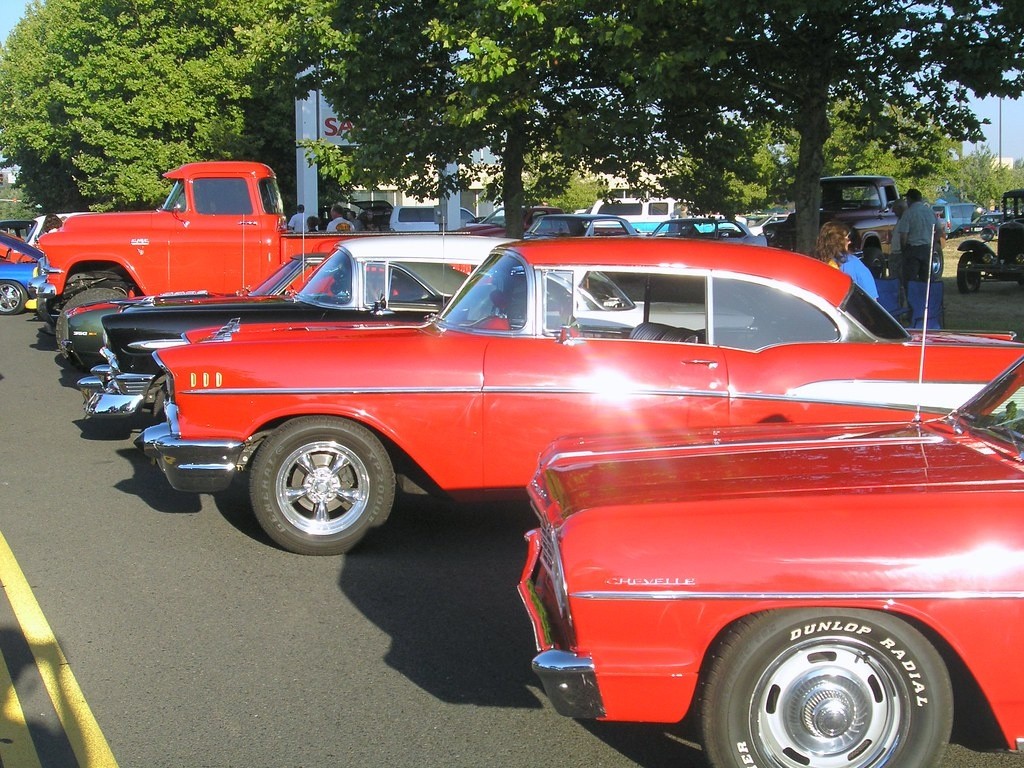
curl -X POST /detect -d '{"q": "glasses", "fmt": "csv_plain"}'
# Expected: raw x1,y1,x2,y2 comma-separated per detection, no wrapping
844,236,850,242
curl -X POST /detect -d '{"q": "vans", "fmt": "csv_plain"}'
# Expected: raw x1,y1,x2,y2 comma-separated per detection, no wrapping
581,196,748,233
348,200,476,232
931,203,984,235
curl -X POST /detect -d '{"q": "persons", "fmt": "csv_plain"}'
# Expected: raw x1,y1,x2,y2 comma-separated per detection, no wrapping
288,205,309,233
735,213,747,225
887,200,907,278
680,206,688,218
307,216,320,232
344,208,380,231
542,202,548,206
898,188,942,311
44,214,62,233
971,207,982,223
814,221,878,303
326,205,355,231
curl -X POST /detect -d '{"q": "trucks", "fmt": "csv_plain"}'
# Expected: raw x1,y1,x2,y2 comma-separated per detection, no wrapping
28,161,470,339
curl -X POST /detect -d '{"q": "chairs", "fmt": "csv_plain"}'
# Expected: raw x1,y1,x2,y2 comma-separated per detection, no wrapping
629,322,672,341
660,327,701,344
874,280,946,329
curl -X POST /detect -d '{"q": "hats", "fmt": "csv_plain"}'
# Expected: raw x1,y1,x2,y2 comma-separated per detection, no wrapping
903,189,923,202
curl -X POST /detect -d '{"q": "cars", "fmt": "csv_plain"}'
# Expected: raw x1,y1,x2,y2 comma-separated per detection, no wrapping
514,360,1024,768
45,234,538,431
749,214,788,236
964,213,1019,241
451,207,566,239
524,214,639,239
762,176,944,282
0,212,100,316
646,219,768,248
132,237,1018,557
